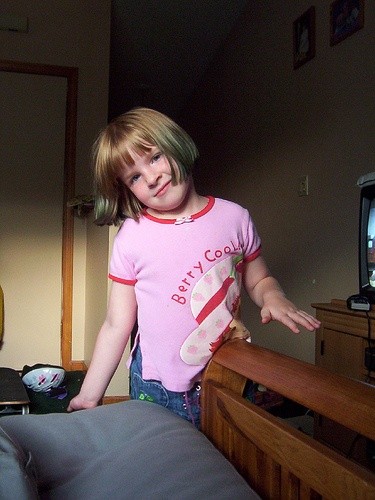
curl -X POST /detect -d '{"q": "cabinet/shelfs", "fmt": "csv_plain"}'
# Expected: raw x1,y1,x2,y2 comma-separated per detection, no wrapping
311,303,375,387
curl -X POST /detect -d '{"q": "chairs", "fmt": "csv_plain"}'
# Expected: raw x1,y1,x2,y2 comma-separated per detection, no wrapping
0,287,30,415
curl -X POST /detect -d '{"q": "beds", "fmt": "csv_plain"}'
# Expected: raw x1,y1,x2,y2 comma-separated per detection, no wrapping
0,339,375,500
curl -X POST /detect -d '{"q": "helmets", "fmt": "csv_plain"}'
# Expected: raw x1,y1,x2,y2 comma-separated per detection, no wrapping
21,364,66,393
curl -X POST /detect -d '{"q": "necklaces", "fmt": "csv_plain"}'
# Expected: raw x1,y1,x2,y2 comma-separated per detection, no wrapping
66,108,322,431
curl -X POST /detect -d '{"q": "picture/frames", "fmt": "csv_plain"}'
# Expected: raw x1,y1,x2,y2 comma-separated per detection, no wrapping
329,0,365,46
291,6,315,69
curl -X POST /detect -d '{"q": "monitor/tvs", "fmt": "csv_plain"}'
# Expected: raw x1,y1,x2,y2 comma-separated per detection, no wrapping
357,183,375,304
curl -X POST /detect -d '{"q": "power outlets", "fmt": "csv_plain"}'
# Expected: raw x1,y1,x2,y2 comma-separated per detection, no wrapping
297,175,308,196
